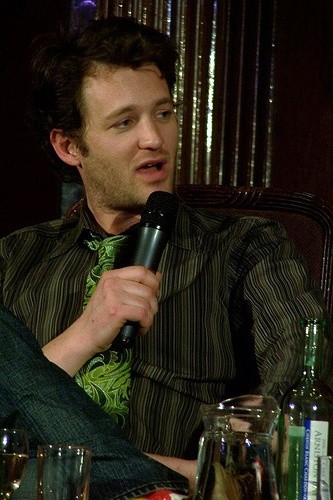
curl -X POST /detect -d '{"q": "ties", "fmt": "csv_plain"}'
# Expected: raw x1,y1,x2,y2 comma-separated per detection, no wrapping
72,231,133,429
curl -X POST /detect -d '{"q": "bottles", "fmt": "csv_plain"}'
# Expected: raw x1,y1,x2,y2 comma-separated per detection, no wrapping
277,317,333,500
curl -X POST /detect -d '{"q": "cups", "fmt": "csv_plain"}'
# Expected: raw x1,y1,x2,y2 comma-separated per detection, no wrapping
36,444,90,500
315,455,333,500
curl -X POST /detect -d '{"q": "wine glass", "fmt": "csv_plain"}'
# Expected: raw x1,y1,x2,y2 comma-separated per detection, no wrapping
0,427,30,500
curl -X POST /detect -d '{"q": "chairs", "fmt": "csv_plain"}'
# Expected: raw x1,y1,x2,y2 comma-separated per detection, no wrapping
61,185,333,317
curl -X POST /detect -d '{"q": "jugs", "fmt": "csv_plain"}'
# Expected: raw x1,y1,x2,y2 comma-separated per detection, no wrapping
194,394,282,500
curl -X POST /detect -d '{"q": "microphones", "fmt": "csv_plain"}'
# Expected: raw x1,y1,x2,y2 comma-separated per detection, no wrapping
112,190,178,348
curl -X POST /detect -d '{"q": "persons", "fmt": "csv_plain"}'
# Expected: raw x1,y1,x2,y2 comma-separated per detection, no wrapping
0,17,330,500
